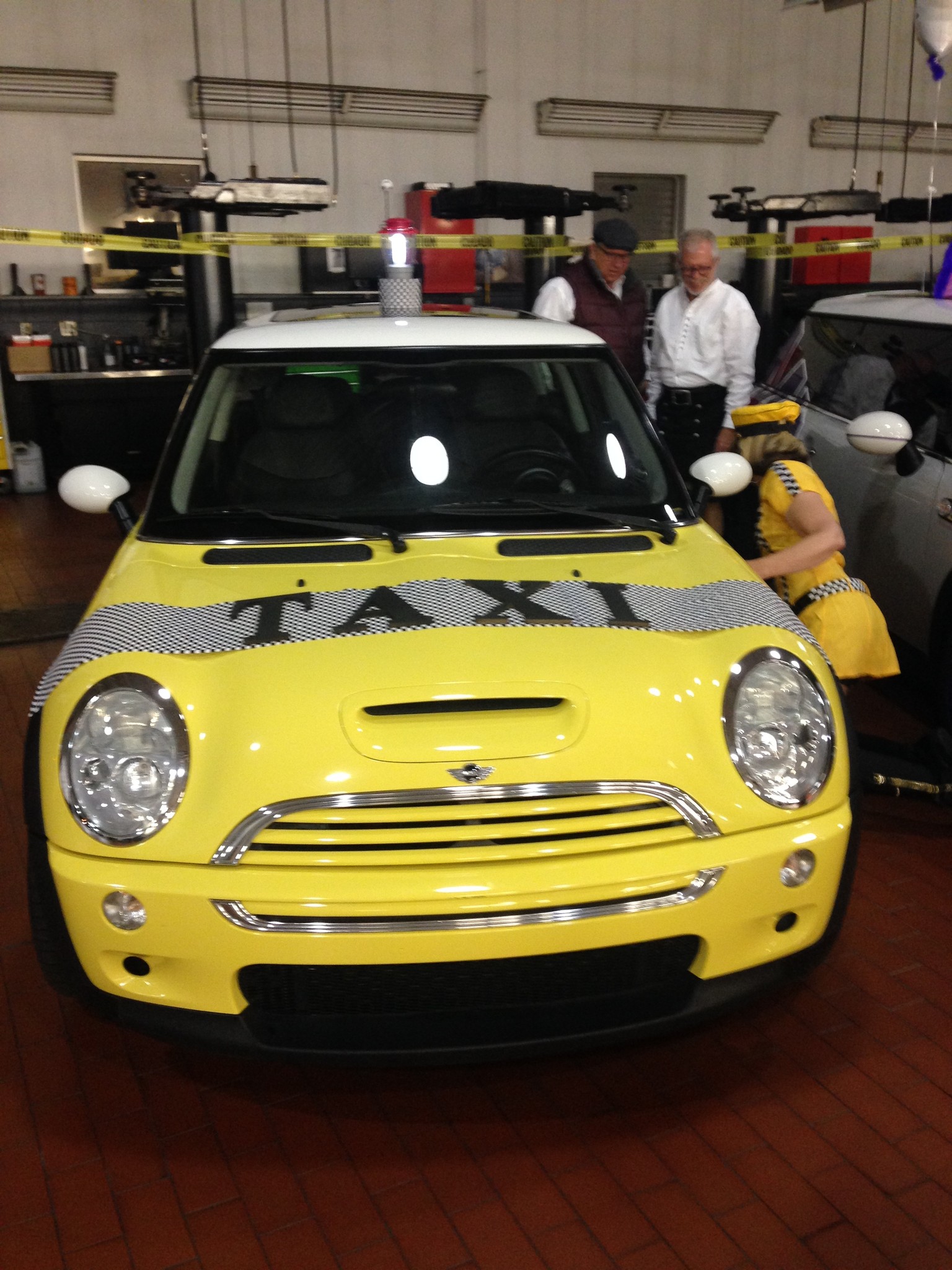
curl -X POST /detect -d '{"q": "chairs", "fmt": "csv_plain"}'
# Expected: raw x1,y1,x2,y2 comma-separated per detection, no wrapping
436,367,583,493
222,370,365,518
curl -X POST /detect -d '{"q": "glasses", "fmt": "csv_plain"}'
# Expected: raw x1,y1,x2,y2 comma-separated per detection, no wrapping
679,261,715,277
595,242,636,261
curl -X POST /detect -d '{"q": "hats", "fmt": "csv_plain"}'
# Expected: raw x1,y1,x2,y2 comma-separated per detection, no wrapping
593,218,638,252
731,398,802,439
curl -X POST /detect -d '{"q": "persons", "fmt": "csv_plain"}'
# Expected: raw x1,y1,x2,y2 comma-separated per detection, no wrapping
823,330,951,460
526,216,653,406
727,396,904,798
640,228,764,539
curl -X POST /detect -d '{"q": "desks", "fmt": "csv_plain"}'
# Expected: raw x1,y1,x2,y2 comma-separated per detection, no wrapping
13,367,196,486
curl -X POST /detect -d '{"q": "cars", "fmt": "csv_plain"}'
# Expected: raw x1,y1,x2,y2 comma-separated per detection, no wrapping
24,307,856,1058
756,290,952,780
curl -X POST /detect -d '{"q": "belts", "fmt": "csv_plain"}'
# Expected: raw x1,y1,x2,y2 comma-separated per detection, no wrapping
660,384,725,406
